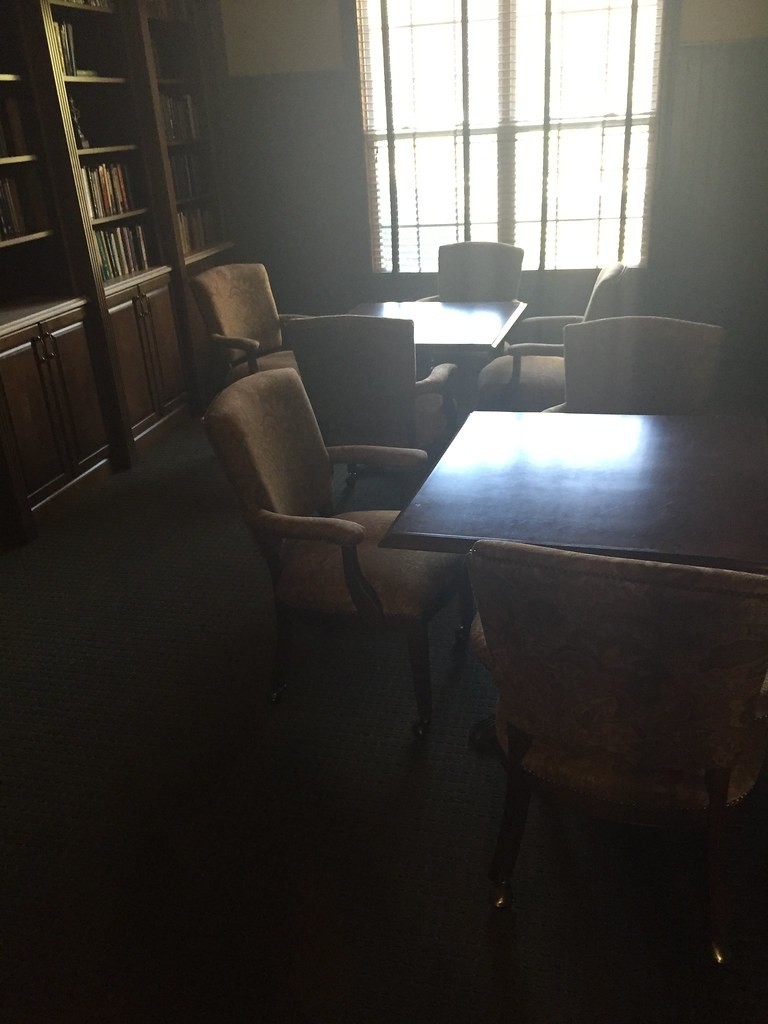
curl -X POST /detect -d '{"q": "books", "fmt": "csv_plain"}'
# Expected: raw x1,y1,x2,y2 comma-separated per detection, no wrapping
0,177,27,237
53,22,76,78
81,162,148,281
159,94,217,254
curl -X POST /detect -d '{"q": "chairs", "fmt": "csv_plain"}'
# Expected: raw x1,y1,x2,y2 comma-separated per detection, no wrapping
282,315,462,488
466,539,768,965
542,316,724,415
203,366,472,760
190,262,314,394
476,261,632,410
413,240,524,384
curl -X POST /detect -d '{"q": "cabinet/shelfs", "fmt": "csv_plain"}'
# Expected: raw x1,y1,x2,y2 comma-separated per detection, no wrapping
0,0,234,554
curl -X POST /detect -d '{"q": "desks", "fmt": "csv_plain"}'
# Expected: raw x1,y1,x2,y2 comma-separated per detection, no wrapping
345,301,529,426
379,408,768,751
